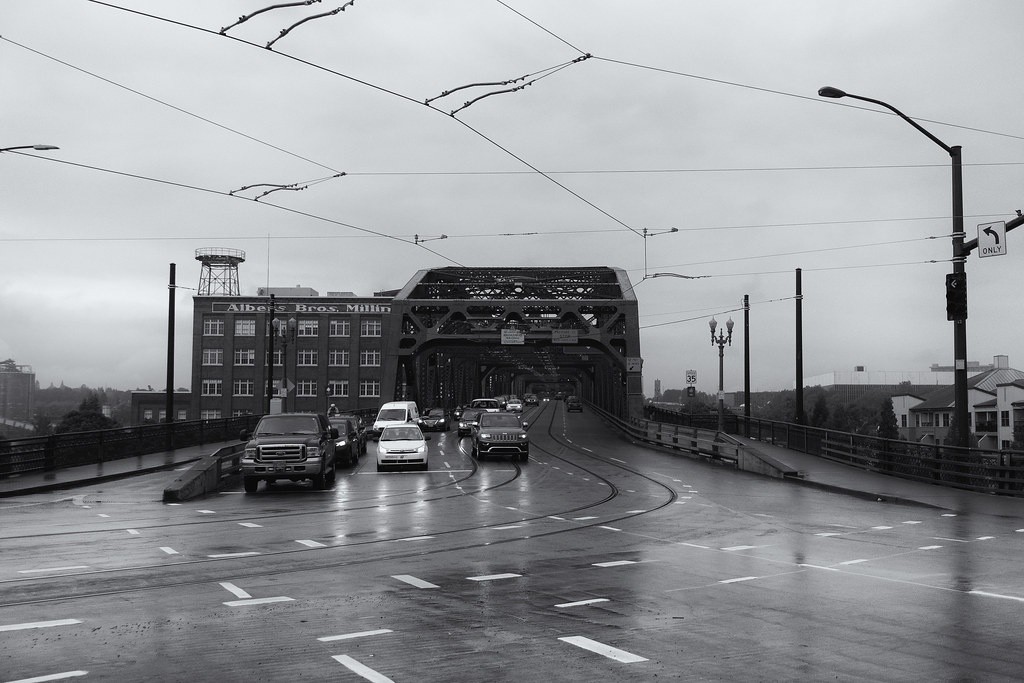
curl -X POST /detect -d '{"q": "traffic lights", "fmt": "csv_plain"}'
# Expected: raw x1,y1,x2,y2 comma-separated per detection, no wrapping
944,273,968,321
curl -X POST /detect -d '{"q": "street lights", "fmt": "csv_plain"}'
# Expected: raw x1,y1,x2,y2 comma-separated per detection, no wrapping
708,314,734,437
817,85,971,490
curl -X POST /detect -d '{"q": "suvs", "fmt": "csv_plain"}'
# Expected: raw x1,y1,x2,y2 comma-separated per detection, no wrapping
467,411,530,461
239,411,339,493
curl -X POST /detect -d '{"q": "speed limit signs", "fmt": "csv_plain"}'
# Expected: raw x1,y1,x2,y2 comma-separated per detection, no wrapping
685,370,697,384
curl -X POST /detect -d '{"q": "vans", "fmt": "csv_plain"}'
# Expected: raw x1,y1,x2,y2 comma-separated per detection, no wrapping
373,401,420,437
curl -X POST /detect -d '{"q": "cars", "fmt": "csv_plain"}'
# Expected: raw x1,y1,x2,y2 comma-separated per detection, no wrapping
420,389,583,434
328,412,368,469
373,424,431,472
